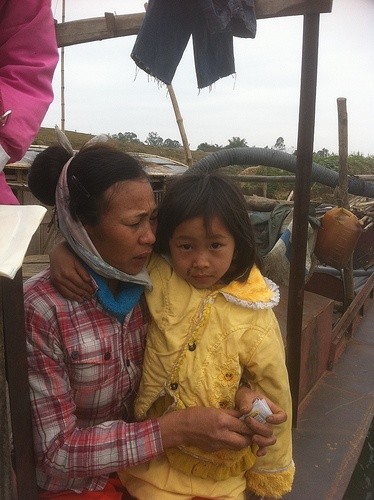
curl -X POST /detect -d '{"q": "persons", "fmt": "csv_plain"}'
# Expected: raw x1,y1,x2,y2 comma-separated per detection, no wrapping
0,0,59,205
23,125,288,500
48,171,296,500
